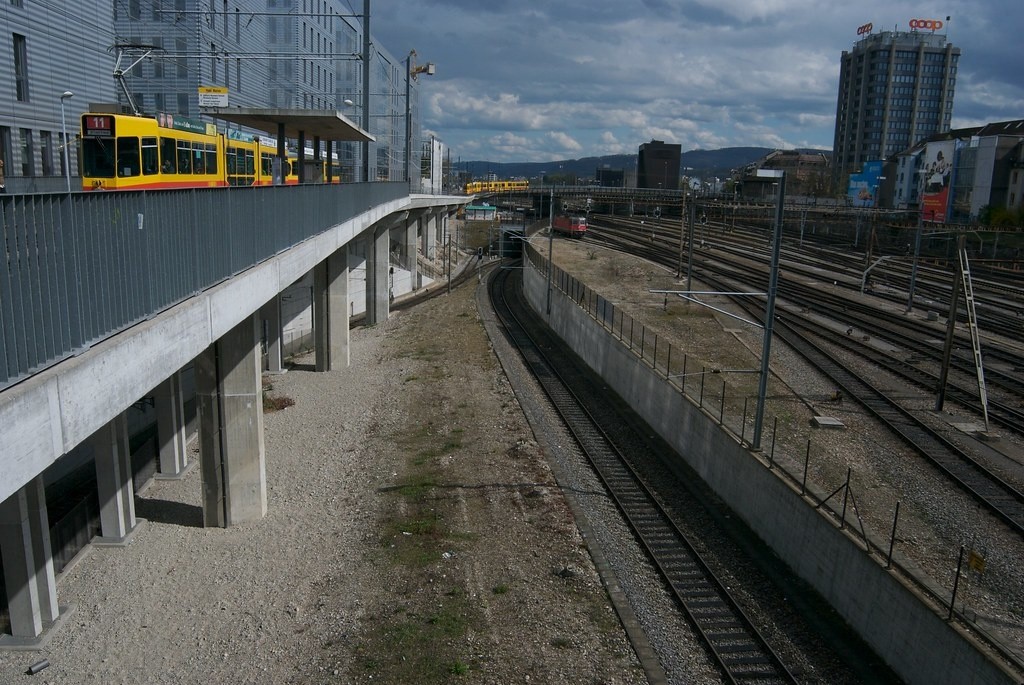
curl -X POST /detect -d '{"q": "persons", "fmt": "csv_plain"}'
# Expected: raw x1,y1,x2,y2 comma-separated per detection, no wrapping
923,151,952,194
162,157,205,174
905,244,911,257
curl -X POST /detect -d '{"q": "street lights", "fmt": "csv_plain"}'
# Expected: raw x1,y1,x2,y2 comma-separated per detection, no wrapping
60,91,73,192
864,175,888,283
905,168,928,310
539,171,547,220
748,168,787,452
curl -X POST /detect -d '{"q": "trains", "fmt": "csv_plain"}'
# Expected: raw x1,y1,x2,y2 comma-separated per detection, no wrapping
458,177,530,196
552,214,588,240
79,44,341,193
